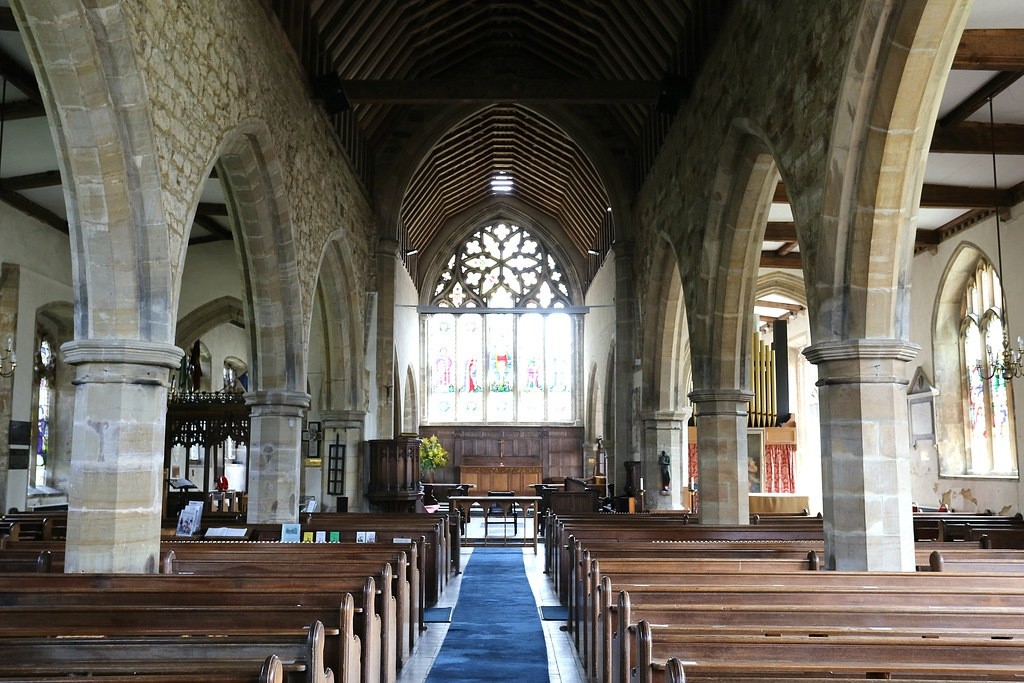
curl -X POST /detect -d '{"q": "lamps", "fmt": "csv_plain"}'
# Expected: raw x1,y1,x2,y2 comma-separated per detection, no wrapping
976,96,1024,380
0,339,18,378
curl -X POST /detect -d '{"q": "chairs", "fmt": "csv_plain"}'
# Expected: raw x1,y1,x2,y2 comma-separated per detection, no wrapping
484,490,517,535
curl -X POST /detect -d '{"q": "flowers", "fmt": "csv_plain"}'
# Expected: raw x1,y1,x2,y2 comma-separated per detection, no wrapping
419,437,450,474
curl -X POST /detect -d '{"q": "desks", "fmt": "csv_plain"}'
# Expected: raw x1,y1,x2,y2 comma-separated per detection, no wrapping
448,495,542,555
749,493,811,513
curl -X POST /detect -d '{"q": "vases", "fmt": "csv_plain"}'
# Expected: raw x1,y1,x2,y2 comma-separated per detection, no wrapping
421,466,434,484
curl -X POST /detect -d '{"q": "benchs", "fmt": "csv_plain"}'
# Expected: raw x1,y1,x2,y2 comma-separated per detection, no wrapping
529,477,1024,683
0,483,475,683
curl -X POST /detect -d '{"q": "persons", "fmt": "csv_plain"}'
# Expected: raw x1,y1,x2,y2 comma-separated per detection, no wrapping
215,473,228,492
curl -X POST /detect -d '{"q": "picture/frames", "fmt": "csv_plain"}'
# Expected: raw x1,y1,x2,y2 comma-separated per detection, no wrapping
747,428,765,495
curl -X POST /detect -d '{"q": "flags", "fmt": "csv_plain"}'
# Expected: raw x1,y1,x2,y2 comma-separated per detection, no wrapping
239,371,248,392
191,339,203,392
